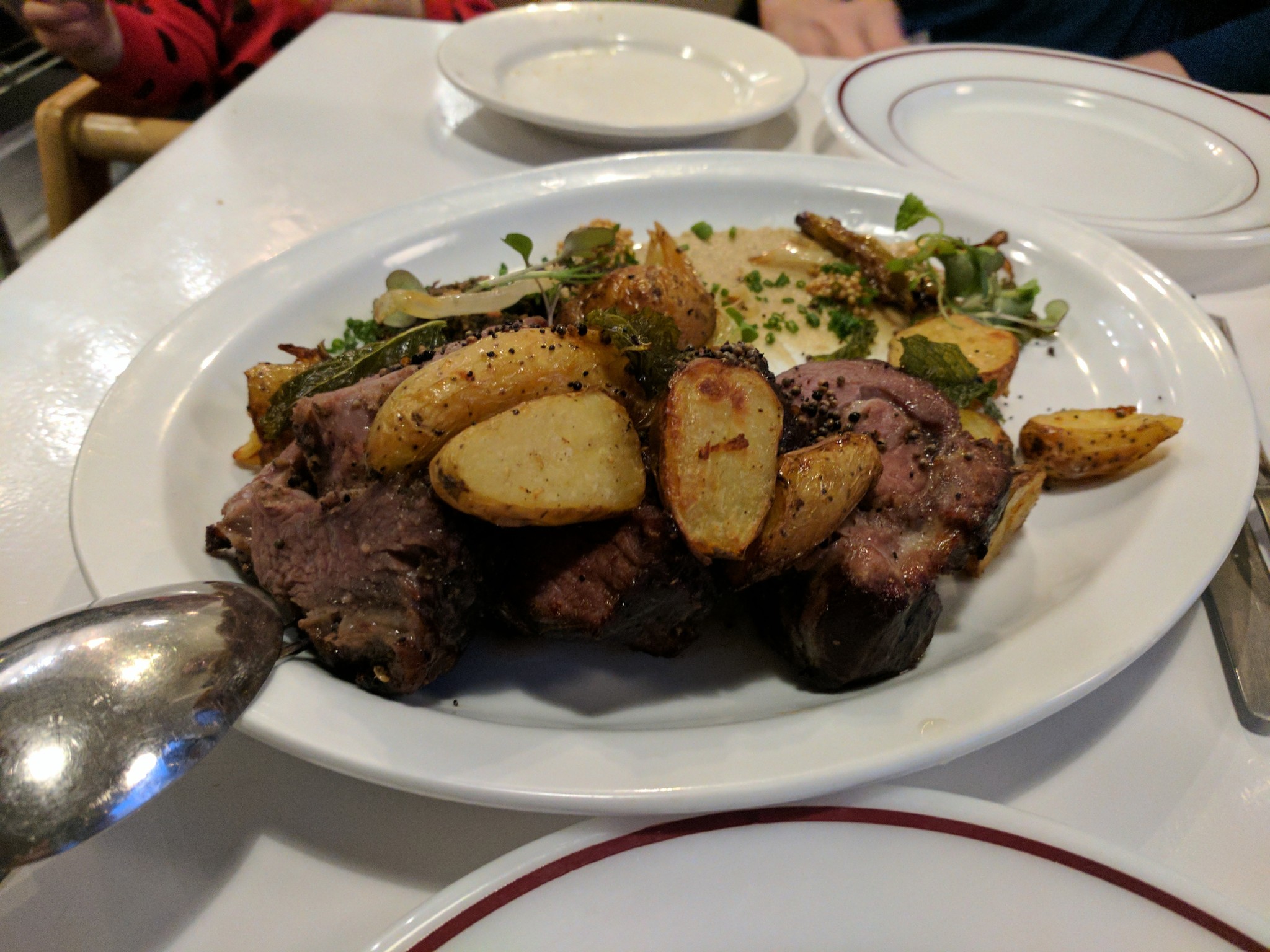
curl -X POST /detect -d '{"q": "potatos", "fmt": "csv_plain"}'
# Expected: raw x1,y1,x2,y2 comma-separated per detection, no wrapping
230,220,1186,582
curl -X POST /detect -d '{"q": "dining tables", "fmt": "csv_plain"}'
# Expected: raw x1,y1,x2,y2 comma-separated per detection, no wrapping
0,10,1270,952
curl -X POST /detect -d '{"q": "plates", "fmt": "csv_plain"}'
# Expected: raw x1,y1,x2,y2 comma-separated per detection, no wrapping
64,145,1263,814
820,40,1270,250
437,1,810,141
364,777,1270,952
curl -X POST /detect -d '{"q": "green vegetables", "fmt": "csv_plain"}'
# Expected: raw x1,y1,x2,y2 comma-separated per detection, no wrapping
256,191,1071,419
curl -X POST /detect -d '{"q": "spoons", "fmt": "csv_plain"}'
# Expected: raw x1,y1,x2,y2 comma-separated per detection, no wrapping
0,578,285,885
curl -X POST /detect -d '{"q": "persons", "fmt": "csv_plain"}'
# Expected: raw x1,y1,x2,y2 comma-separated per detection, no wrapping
22,0,504,123
756,1,1270,98
